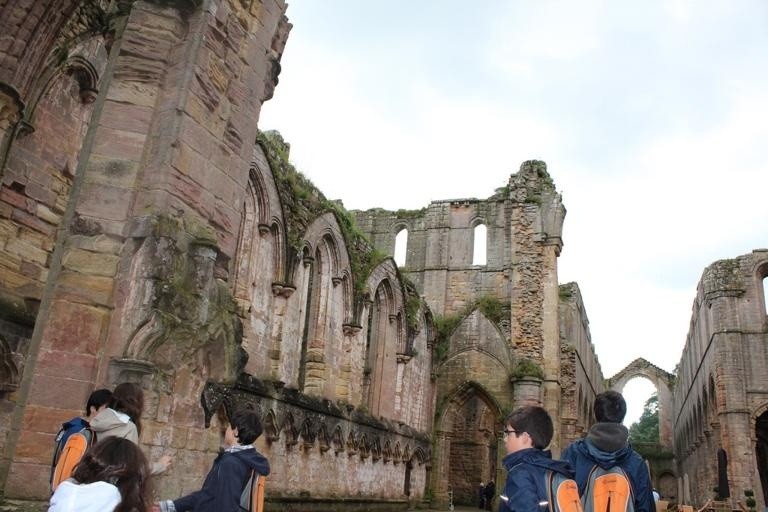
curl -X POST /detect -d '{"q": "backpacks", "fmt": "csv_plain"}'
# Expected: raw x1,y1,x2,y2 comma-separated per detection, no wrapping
580,462,637,511
545,468,583,511
239,469,265,512
50,427,97,495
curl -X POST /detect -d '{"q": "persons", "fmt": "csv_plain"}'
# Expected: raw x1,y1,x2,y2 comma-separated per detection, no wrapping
496,405,576,512
89,382,173,477
47,435,156,512
479,480,495,511
145,409,270,512
561,390,656,512
50,388,112,496
652,488,661,507
